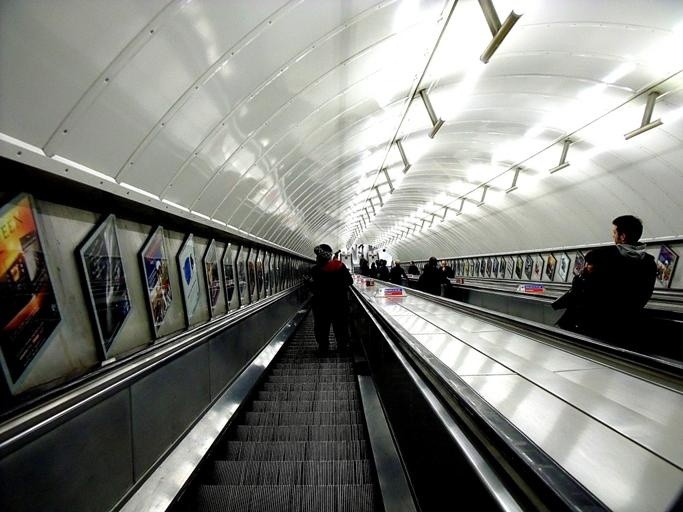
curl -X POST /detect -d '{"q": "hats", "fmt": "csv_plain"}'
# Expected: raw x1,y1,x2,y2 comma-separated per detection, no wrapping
313,244,332,259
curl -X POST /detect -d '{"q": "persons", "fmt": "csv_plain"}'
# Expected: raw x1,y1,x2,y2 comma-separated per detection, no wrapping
571,214,657,344
357,254,454,299
551,248,605,331
305,244,353,356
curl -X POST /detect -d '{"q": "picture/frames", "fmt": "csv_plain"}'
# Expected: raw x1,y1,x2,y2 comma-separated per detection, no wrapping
447,245,679,290
